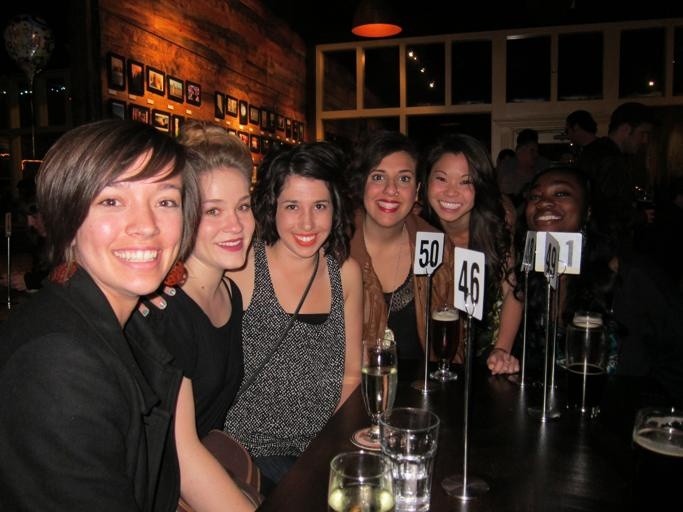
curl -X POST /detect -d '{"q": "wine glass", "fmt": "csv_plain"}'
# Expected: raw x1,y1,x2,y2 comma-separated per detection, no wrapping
355,337,397,443
428,305,459,384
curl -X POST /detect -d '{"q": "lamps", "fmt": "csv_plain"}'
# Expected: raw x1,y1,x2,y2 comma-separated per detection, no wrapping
348,0,405,45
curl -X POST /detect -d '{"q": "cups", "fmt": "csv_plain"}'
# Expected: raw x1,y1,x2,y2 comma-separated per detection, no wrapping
377,408,440,509
326,450,395,512
558,308,682,511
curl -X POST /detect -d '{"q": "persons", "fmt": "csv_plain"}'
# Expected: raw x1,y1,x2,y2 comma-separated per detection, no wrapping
418,129,527,376
122,124,264,512
0,113,199,511
137,138,366,503
0,169,62,292
346,130,462,385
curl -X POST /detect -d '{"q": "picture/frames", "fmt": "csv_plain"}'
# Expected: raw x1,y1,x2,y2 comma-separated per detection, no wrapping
99,49,307,192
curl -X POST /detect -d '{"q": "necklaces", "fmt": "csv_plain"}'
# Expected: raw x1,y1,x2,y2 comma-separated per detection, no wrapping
364,223,406,352
